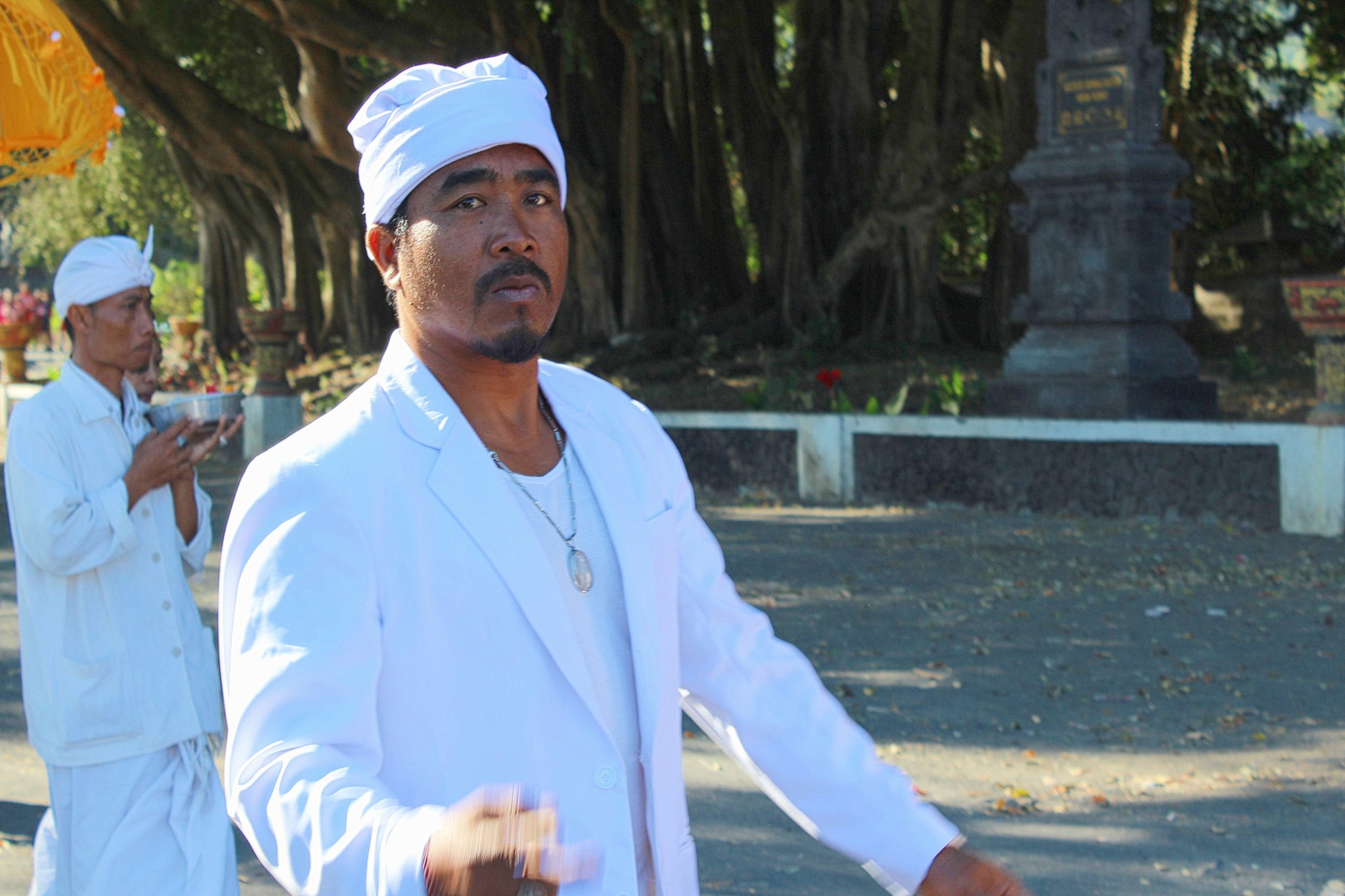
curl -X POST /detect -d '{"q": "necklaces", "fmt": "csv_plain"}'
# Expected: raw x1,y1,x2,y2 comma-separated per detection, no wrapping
485,393,592,589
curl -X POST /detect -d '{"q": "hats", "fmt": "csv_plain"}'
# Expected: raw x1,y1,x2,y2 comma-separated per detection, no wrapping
348,53,567,226
53,225,155,319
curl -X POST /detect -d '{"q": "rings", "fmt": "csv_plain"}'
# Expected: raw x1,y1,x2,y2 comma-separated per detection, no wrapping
220,435,228,446
177,433,187,447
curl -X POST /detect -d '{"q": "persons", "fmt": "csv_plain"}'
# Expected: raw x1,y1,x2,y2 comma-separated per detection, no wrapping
6,224,237,896
219,54,1026,896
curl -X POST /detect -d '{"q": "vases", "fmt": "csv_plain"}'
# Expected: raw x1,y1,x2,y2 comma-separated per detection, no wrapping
0,324,36,384
237,310,307,397
1281,277,1344,425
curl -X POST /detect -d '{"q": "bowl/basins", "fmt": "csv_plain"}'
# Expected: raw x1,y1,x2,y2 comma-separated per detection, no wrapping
142,392,246,434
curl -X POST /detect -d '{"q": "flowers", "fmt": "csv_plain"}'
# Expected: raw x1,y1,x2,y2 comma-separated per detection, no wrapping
0,289,51,325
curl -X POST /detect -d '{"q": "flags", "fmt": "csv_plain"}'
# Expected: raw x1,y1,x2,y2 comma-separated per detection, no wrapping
0,0,124,188
